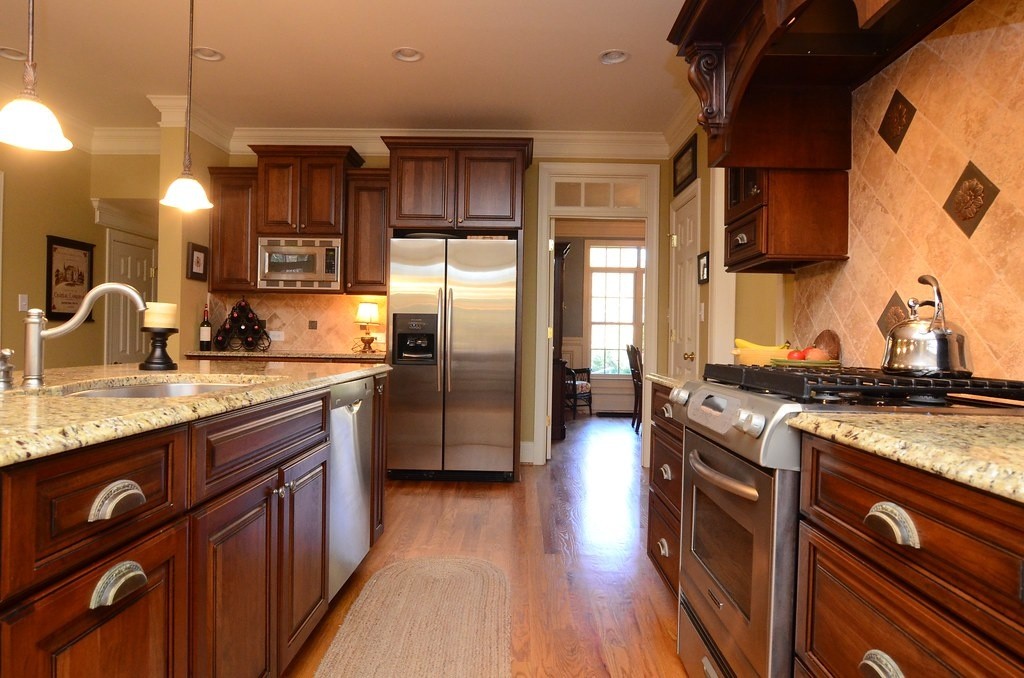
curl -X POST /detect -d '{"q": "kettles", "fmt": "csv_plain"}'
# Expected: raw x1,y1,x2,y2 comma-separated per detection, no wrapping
881,275,951,376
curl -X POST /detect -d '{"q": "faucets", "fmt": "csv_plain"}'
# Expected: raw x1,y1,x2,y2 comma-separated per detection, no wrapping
23,281,149,387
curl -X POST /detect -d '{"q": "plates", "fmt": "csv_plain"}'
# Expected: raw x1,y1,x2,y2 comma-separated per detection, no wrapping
771,359,841,368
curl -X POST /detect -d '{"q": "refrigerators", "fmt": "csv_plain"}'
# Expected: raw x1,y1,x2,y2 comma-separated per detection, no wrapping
386,238,517,482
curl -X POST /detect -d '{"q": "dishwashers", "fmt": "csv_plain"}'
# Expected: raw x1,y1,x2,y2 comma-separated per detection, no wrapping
326,376,375,603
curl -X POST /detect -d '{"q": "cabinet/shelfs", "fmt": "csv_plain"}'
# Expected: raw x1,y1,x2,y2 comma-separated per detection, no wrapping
204,136,535,295
793,434,1024,678
726,168,849,274
647,382,684,602
0,373,329,678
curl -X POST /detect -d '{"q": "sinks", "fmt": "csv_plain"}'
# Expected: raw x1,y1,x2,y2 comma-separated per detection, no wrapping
42,374,277,399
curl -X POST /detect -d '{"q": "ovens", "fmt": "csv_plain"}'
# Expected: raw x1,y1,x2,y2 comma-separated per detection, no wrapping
676,426,801,678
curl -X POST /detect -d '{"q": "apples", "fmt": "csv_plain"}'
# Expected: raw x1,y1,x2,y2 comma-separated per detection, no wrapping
787,346,814,360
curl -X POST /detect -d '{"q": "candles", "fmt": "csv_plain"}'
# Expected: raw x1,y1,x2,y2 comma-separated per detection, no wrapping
144,302,178,329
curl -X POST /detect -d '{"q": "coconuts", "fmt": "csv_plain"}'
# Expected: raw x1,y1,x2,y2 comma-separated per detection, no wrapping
813,329,840,360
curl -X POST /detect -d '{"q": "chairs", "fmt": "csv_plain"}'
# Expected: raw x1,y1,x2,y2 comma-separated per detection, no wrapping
565,368,593,420
627,345,643,432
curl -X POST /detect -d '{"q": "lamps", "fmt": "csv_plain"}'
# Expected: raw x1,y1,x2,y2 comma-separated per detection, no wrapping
0,0,72,152
159,0,214,212
354,301,380,353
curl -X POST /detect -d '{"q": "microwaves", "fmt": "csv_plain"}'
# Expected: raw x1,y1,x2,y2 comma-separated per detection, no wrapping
258,238,341,291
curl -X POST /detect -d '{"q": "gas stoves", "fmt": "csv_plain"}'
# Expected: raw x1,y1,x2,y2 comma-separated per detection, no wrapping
668,364,1024,471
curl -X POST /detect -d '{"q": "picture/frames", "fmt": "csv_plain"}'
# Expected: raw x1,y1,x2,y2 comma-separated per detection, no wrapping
186,242,209,281
697,250,709,284
673,133,697,196
45,235,96,322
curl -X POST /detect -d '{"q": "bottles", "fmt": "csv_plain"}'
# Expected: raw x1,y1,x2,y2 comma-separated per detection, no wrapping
199,304,213,352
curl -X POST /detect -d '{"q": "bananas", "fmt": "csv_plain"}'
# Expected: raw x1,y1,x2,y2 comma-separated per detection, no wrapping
734,339,791,350
